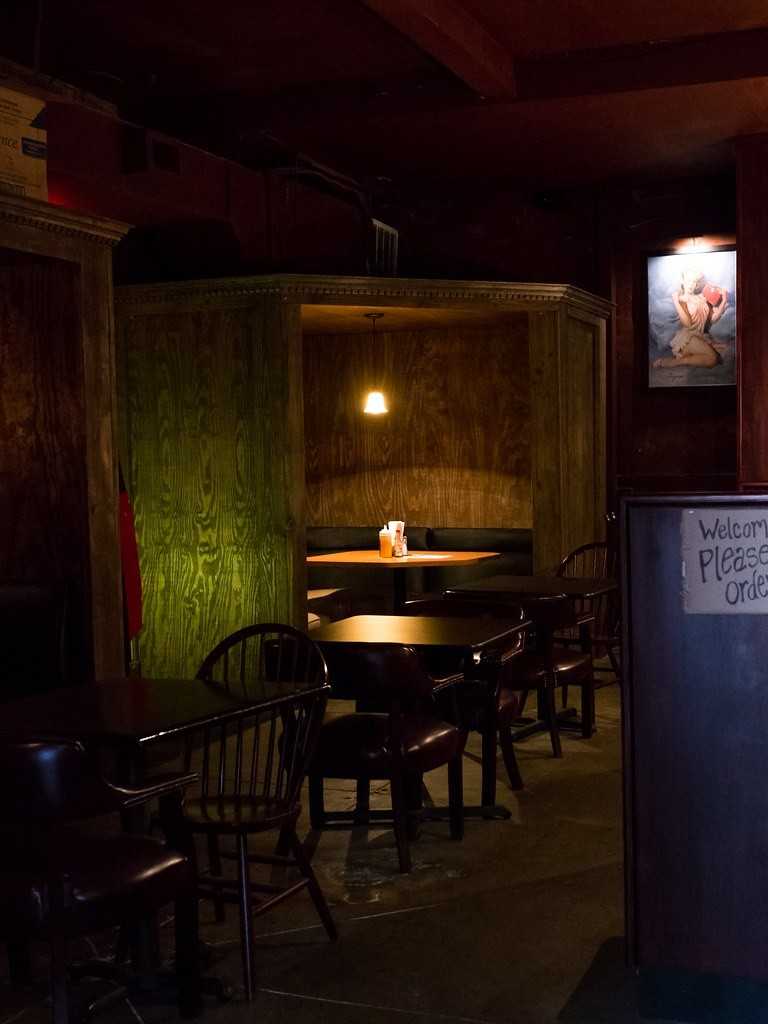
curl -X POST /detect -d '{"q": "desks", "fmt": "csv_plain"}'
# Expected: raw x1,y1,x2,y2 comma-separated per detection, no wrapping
0,680,329,1019
293,617,531,841
444,576,617,737
306,549,502,615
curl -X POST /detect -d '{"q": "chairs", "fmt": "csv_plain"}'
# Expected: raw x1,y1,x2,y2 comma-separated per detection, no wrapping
0,738,198,1024
554,541,623,698
260,639,472,870
148,623,340,942
449,593,595,738
397,601,533,791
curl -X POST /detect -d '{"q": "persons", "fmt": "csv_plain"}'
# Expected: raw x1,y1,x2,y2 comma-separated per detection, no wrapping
651,265,728,369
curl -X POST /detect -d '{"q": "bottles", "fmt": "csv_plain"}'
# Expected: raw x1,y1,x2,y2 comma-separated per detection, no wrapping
394,530,403,556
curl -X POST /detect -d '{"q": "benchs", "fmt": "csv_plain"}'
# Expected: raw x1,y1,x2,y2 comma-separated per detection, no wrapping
308,514,536,592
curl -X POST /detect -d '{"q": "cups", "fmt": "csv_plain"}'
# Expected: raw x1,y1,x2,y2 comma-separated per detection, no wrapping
379,524,393,558
388,521,405,555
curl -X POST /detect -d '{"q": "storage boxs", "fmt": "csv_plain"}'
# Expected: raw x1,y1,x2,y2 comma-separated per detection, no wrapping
0,84,50,201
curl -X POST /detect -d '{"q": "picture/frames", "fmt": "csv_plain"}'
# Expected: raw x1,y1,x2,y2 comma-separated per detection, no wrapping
646,245,740,391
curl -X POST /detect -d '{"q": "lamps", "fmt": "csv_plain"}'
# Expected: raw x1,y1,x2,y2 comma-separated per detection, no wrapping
360,311,389,415
